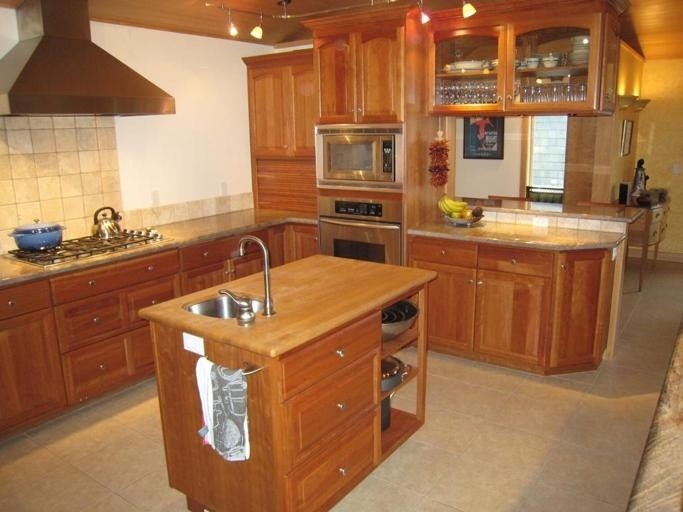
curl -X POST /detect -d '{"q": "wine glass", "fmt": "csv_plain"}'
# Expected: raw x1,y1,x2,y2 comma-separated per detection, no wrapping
439,80,496,104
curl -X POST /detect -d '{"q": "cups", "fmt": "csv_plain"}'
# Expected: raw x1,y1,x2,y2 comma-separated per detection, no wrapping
490,58,497,69
520,83,585,103
514,57,560,68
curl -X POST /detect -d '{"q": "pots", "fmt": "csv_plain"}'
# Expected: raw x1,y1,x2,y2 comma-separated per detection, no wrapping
8,219,66,250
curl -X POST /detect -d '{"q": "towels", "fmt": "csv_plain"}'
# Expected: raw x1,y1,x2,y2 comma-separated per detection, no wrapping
192,357,251,463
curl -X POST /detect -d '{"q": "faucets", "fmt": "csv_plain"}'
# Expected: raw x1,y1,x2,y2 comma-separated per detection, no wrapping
236,233,272,316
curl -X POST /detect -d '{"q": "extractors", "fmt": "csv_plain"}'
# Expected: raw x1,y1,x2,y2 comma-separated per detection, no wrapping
0,0,177,119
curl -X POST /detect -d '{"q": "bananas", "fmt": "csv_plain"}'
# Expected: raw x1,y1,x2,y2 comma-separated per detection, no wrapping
437,193,467,215
449,211,470,219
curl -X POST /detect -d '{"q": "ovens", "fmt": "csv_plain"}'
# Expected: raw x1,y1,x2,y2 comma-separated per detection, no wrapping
318,196,402,267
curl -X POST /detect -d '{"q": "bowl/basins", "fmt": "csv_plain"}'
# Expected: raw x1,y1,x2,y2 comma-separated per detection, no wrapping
381,299,420,340
567,34,590,65
381,356,411,393
442,212,484,226
444,60,489,72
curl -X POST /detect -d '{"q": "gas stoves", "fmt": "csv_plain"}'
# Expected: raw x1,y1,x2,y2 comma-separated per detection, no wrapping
8,229,157,264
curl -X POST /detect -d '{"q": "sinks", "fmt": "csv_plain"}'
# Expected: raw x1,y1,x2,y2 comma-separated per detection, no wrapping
184,293,266,320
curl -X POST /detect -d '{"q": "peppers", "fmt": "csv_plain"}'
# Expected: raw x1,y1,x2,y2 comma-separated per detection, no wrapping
428,138,451,189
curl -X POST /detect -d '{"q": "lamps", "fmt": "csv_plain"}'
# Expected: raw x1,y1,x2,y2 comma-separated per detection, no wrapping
617,93,651,113
203,0,479,40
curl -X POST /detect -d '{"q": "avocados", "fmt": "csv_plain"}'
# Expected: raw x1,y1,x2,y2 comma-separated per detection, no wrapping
472,206,484,217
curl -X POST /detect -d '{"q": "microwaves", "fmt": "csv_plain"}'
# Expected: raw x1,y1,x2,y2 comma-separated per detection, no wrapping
315,122,404,191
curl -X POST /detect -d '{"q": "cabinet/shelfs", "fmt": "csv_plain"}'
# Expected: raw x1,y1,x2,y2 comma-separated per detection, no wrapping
180,224,284,294
423,0,627,118
241,48,317,215
614,196,671,294
408,234,554,374
285,223,319,263
280,310,378,512
378,285,425,465
0,278,66,440
550,247,615,376
50,248,180,408
299,5,447,125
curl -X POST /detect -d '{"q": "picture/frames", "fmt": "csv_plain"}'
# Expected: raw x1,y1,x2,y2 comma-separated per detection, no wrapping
462,116,505,160
620,119,634,156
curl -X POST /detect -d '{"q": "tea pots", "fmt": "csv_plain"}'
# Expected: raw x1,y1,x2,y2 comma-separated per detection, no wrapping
90,206,124,238
